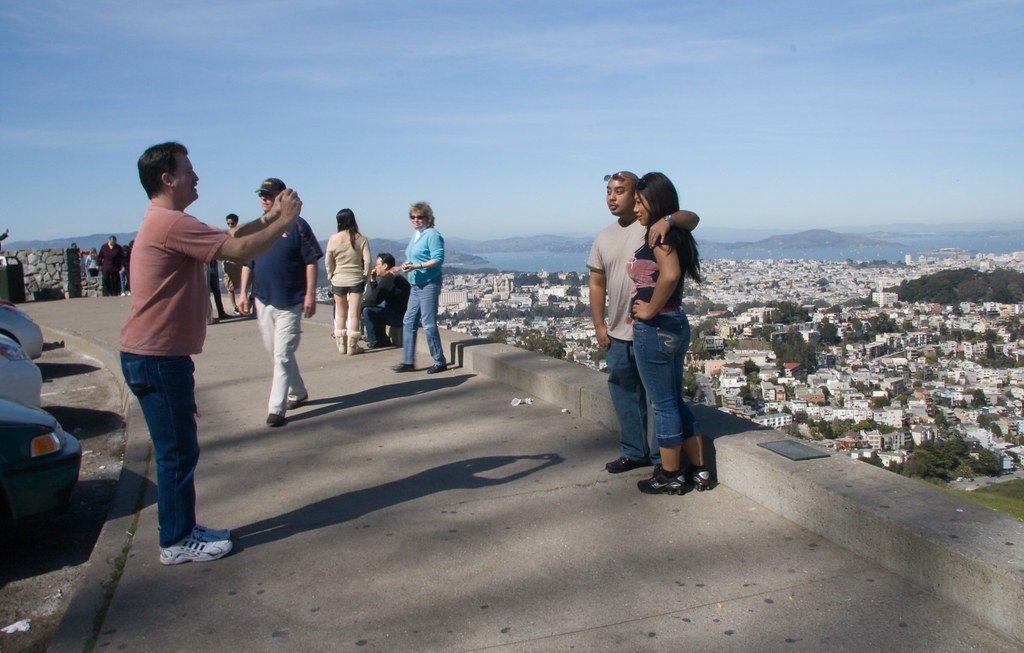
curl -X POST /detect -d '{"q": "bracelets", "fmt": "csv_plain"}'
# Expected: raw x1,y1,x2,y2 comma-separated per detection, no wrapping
420,263,422,268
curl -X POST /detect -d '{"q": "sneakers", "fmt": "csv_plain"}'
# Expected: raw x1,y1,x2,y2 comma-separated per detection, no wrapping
637,470,685,495
192,525,230,539
685,465,711,491
160,534,233,565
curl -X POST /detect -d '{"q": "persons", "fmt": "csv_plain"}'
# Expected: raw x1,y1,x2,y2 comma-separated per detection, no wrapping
119,240,135,296
238,179,322,426
361,253,412,348
0,228,9,253
587,171,700,476
324,208,372,356
221,214,243,317
626,171,712,496
209,259,230,319
96,235,124,296
70,243,80,253
85,247,99,277
119,140,303,566
389,200,447,373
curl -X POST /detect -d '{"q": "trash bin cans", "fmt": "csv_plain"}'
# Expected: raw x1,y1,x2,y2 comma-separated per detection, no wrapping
0,256,26,303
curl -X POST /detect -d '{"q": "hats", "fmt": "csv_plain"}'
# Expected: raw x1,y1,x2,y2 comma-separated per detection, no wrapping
255,178,286,192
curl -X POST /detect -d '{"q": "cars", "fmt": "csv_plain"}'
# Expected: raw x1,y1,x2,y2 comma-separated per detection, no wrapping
0,296,44,361
0,331,43,409
0,395,83,562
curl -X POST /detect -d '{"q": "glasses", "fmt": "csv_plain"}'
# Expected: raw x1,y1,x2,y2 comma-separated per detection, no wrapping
227,223,234,226
604,173,634,181
411,216,427,219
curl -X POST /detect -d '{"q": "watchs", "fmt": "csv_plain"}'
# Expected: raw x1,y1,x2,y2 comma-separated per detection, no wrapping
664,215,673,227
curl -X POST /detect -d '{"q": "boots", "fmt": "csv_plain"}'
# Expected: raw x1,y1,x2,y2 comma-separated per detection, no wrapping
347,330,365,355
334,329,348,353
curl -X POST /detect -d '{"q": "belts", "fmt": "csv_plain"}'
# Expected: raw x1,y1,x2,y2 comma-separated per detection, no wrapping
658,305,684,315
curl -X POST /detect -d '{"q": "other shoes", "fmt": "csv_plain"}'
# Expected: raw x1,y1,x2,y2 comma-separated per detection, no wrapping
286,394,307,409
121,293,125,296
654,464,662,475
219,312,234,319
126,291,130,296
367,337,391,348
606,457,653,473
391,364,414,372
233,307,241,315
206,318,219,324
267,414,284,425
427,364,447,374
